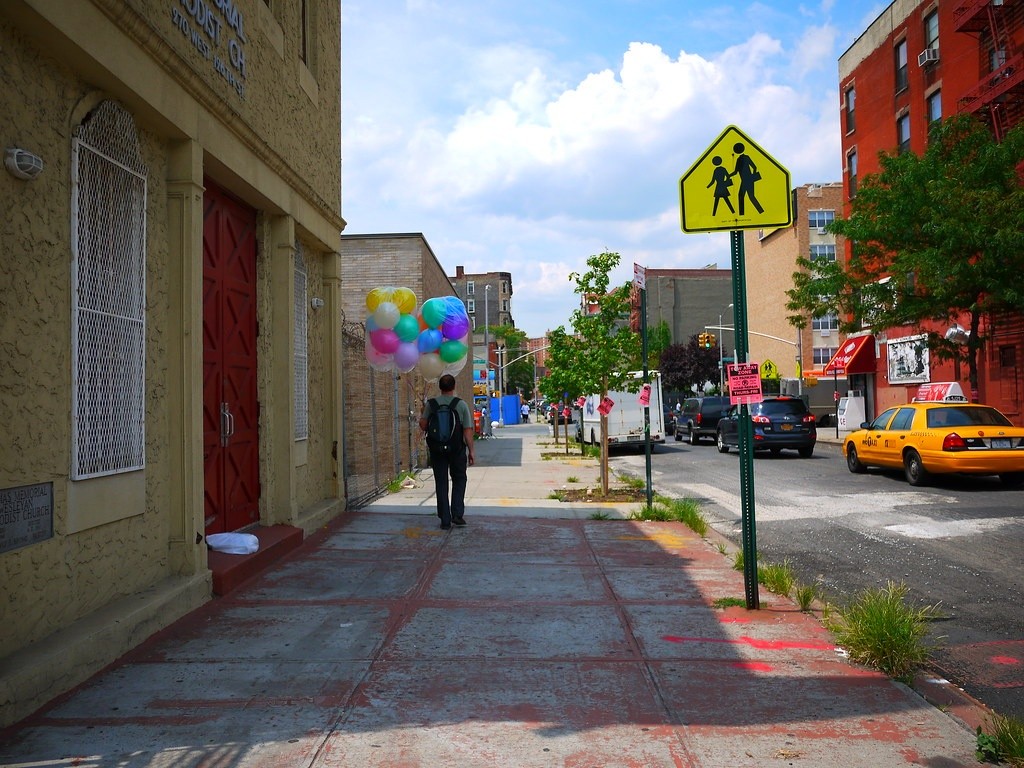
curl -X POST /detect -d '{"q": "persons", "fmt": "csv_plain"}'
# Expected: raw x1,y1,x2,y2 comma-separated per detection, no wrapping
482,405,487,416
418,374,475,530
676,402,682,411
521,403,530,423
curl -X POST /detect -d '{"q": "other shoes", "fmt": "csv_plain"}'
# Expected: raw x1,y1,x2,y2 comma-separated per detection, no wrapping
440,521,451,529
452,516,466,525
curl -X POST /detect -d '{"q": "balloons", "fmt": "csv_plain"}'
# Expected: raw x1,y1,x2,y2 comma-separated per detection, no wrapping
369,329,399,354
366,315,379,331
417,314,428,332
390,287,417,314
421,321,444,354
374,302,400,329
419,353,444,378
443,296,466,316
442,316,469,340
393,313,420,343
417,328,442,353
395,343,419,373
366,288,391,313
422,298,446,329
439,340,468,363
365,344,394,373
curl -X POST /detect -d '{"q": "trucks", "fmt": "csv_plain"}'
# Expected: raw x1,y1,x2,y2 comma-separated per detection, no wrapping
574,370,666,455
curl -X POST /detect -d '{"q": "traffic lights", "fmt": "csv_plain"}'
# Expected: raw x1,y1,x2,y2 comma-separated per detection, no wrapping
698,333,705,348
711,334,716,347
704,334,711,348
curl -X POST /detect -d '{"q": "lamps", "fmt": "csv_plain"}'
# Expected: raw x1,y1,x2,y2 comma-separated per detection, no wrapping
312,297,325,309
4,148,50,183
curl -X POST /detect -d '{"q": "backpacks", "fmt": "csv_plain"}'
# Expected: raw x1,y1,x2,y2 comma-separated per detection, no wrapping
426,397,462,455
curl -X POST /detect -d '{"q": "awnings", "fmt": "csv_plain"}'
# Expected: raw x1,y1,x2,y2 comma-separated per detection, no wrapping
822,328,877,376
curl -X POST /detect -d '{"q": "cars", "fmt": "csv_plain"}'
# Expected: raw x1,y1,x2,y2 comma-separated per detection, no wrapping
662,403,676,436
527,397,575,426
843,381,1024,490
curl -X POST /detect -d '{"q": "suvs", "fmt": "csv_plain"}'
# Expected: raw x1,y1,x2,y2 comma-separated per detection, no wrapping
672,395,735,445
716,393,817,459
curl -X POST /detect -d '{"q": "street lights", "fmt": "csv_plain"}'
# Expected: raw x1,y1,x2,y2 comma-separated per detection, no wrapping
717,304,734,397
